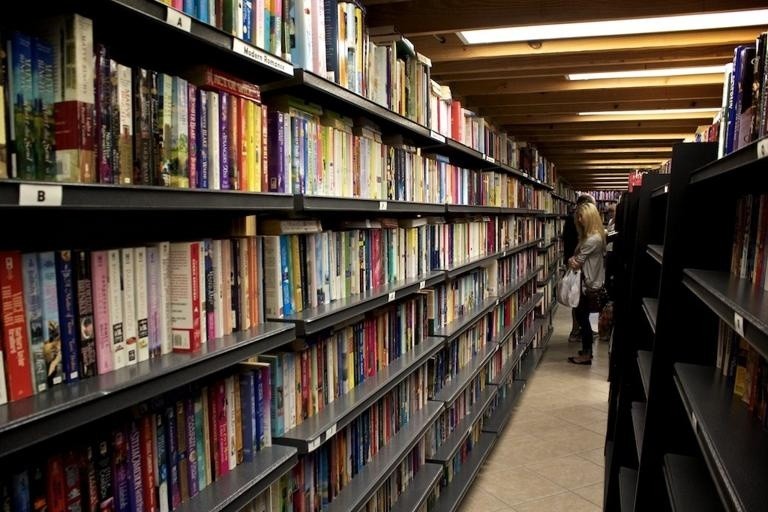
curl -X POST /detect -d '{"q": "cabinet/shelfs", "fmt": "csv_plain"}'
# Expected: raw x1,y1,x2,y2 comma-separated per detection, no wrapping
0,1,578,512
604,134,767,512
573,190,620,228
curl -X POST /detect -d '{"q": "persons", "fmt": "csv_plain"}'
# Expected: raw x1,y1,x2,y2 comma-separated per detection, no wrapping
567,204,604,366
604,203,616,255
563,194,600,342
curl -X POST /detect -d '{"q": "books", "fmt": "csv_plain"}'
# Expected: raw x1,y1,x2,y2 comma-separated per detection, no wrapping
576,191,623,227
0,0,575,512
627,112,722,193
717,32,767,423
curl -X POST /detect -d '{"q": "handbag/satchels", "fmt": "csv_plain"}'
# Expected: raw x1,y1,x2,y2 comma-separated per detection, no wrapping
598,302,614,340
587,288,608,312
557,272,580,308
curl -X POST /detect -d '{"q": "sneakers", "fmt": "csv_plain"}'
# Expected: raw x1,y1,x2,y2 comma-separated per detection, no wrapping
568,351,593,364
568,333,582,342
